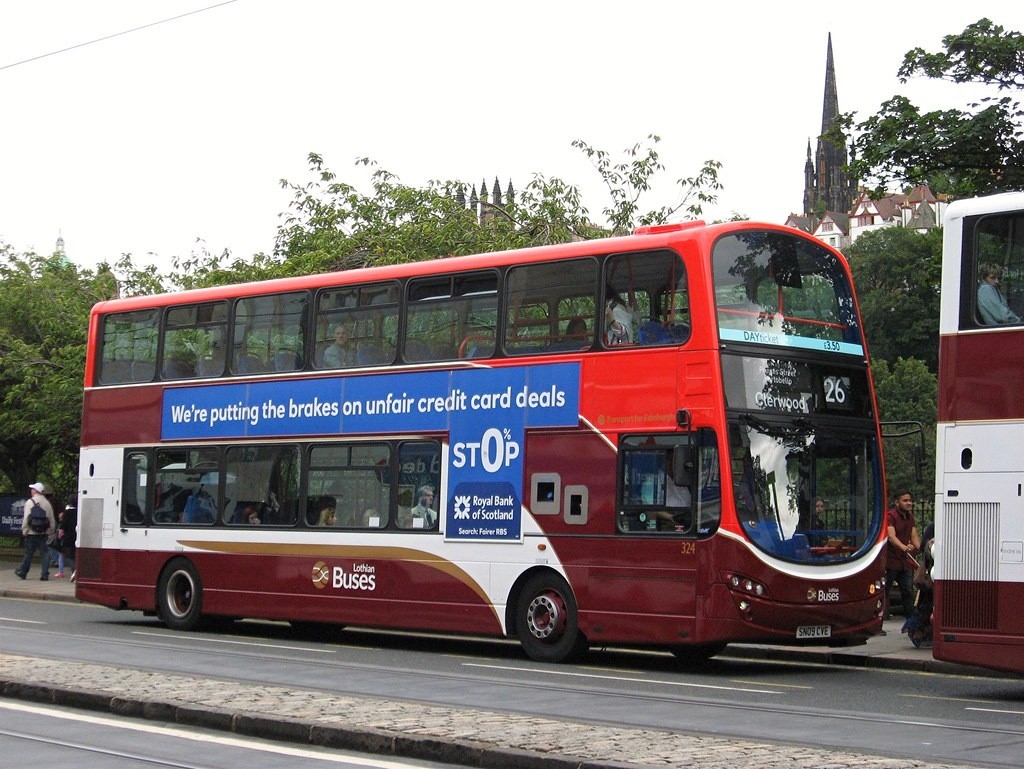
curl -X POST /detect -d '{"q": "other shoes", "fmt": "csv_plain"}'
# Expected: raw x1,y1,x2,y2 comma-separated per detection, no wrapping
55,573,64,578
40,577,48,581
14,570,26,579
878,630,886,636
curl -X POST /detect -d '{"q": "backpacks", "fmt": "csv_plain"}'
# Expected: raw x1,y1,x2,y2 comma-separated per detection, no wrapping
28,498,50,533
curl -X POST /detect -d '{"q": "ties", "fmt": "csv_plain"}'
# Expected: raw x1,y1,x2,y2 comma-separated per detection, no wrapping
425,510,432,527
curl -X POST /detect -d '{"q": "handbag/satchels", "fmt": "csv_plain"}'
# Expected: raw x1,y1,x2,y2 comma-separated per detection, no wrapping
45,528,62,552
913,554,933,593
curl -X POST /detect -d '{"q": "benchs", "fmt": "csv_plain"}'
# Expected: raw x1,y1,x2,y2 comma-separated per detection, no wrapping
973,286,1024,328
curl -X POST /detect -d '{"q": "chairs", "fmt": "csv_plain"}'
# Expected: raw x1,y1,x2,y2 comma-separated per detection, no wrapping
101,321,690,386
126,504,319,525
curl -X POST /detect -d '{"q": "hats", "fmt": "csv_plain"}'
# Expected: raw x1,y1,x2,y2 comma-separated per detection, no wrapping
29,482,44,494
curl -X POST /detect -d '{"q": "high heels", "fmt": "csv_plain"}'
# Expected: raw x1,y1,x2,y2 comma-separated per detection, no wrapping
902,621,921,649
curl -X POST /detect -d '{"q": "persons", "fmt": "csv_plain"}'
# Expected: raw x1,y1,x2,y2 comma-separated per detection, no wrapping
977,262,1021,326
580,282,634,351
173,468,262,524
816,497,828,547
879,490,920,635
559,319,588,351
641,448,695,531
56,495,78,582
310,495,382,526
323,324,357,367
411,486,437,528
913,522,934,625
15,482,55,581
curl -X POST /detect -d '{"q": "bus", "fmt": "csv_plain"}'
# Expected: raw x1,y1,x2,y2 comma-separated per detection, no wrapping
70,216,933,666
928,187,1024,682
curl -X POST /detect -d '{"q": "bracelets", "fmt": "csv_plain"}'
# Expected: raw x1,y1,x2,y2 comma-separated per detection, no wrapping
609,319,615,326
